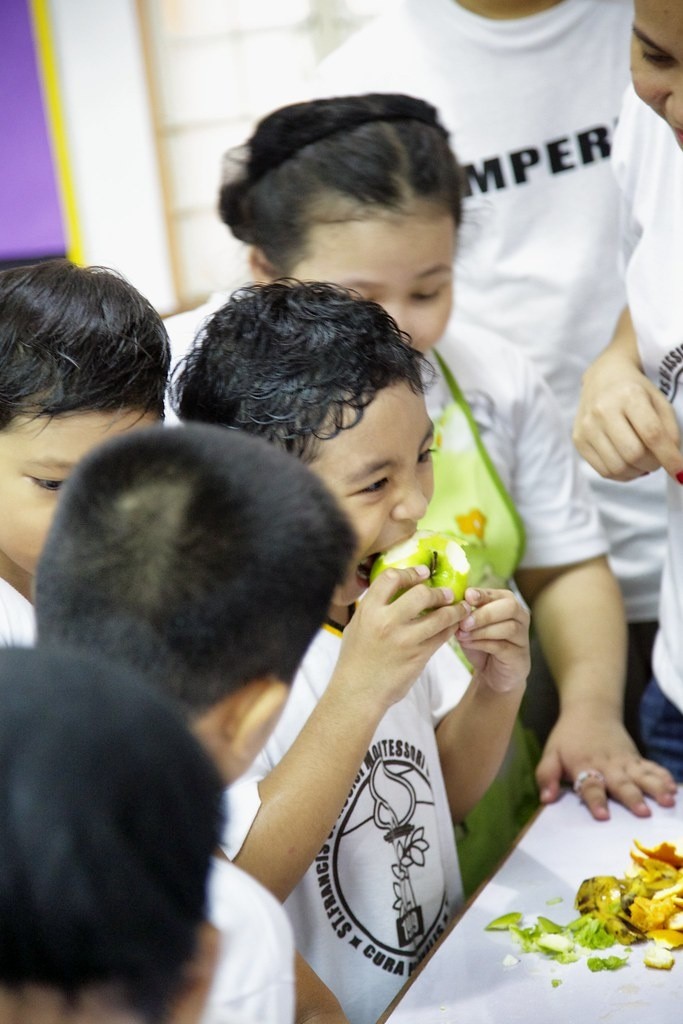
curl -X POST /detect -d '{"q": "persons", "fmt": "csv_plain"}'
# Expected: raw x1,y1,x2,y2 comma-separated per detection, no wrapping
570,0,683,783
277,0,668,733
1,257,172,649
174,282,533,1023
218,92,677,896
36,423,359,1024
0,644,228,1023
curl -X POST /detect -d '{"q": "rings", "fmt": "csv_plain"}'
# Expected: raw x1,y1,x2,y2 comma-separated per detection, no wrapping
574,771,608,792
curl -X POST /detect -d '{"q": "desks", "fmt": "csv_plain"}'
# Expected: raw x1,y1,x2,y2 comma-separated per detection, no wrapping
374,781,683,1024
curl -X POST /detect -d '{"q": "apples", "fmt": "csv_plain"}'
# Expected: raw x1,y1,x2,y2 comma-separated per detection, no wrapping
368,530,471,615
